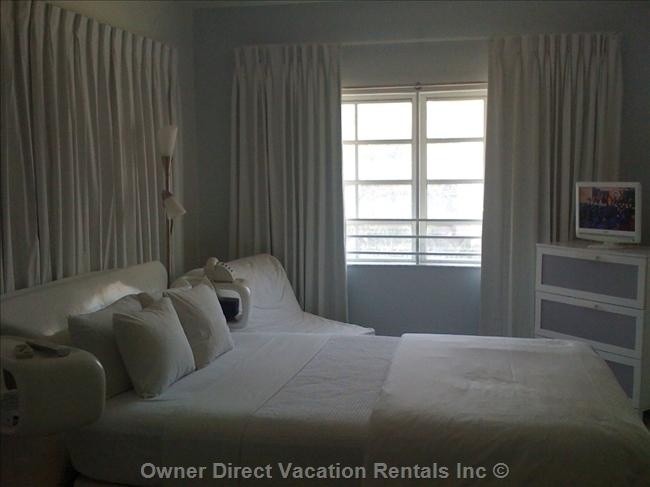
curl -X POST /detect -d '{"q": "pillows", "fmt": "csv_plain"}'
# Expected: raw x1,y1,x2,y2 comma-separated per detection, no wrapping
112,297,196,398
68,293,154,399
162,277,192,296
168,274,233,370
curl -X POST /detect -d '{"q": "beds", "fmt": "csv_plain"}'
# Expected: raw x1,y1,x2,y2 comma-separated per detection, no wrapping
1,262,650,487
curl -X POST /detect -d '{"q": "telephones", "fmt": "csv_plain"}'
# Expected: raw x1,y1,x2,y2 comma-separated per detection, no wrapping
204,257,237,283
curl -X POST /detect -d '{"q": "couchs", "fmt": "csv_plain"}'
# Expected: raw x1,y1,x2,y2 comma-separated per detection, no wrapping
224,254,375,334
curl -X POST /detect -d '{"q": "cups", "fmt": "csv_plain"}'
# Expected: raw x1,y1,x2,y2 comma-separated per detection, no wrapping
219,297,239,317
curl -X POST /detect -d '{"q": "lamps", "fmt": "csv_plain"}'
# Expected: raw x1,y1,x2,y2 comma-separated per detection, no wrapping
155,125,185,289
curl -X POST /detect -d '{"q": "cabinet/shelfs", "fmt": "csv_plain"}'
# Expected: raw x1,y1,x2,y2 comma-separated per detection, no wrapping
534,239,650,419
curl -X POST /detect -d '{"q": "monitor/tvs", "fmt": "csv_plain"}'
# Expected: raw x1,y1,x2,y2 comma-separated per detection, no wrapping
574,181,642,248
